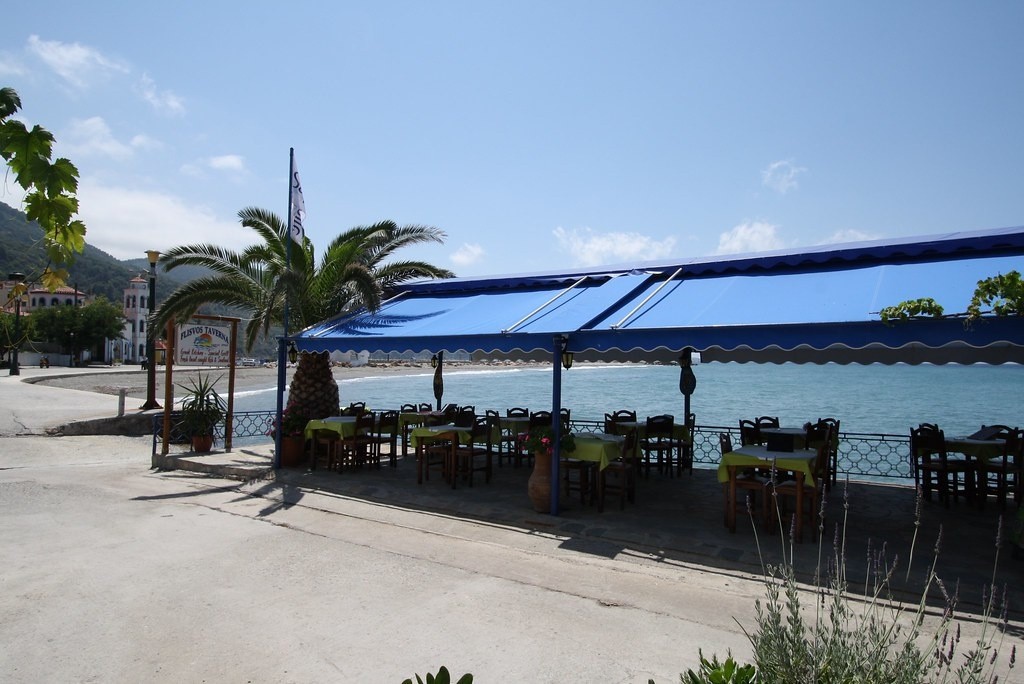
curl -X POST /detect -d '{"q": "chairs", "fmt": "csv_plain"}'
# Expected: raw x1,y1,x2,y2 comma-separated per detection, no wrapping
326,403,366,467
364,411,399,470
911,423,1024,515
401,400,695,513
335,412,376,474
310,408,348,466
720,416,840,542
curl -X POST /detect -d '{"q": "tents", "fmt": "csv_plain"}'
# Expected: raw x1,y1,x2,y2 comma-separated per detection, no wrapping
274,231,1024,514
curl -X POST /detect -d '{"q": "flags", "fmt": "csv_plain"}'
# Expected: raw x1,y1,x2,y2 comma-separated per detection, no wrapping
288,153,307,248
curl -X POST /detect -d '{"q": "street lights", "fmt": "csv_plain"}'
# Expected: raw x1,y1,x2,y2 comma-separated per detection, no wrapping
139,249,164,411
9,272,26,375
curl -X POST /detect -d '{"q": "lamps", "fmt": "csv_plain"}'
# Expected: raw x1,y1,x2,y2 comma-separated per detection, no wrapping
562,352,573,370
431,354,438,368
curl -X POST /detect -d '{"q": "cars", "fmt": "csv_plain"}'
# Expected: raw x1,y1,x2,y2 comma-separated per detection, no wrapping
243,359,256,366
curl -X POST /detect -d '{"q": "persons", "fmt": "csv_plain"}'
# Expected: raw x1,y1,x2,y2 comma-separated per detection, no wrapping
141,355,148,370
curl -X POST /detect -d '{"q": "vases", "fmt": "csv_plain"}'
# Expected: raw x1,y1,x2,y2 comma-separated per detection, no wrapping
527,452,553,512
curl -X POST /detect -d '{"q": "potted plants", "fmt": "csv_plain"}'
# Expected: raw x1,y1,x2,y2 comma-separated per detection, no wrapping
281,438,305,464
169,369,230,452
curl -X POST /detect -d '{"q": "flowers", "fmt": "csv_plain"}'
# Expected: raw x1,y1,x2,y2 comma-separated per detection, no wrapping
514,429,576,454
264,399,308,442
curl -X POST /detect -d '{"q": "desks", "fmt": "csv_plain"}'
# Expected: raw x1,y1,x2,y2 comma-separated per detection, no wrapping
401,412,448,458
760,428,807,449
305,415,399,474
557,432,644,514
499,417,550,464
612,422,690,479
717,444,818,544
918,436,1018,520
411,425,501,489
